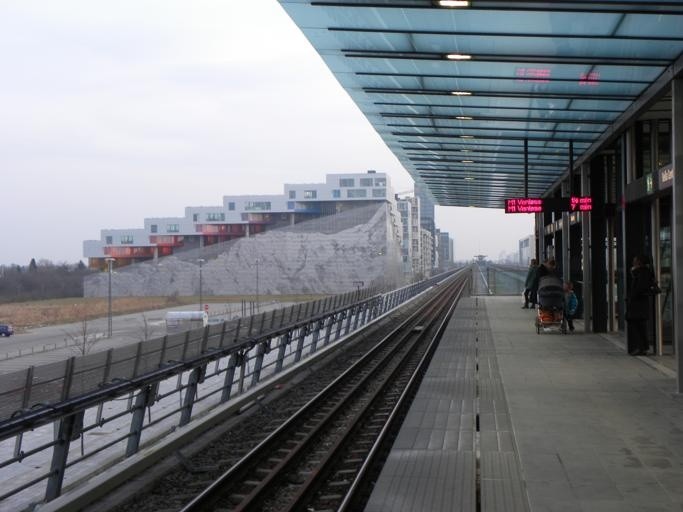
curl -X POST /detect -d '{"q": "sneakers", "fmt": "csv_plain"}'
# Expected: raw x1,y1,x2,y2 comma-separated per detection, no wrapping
543,328,576,335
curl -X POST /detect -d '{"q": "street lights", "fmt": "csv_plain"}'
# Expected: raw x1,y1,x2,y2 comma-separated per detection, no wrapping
196,258,206,311
105,258,116,337
252,259,260,308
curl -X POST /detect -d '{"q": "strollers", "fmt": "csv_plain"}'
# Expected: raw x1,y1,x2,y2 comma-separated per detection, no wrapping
536,276,569,336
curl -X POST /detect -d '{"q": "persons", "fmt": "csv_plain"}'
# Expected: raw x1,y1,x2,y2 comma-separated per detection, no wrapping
521,258,537,307
624,252,655,358
526,260,555,306
559,280,579,333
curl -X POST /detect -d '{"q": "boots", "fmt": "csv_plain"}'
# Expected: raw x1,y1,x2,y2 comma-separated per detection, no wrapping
521,302,536,310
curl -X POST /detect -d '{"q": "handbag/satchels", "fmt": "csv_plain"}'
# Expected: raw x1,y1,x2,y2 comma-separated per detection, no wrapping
521,288,526,305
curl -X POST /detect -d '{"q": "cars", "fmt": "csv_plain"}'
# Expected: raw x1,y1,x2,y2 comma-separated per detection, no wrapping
0,326,12,337
